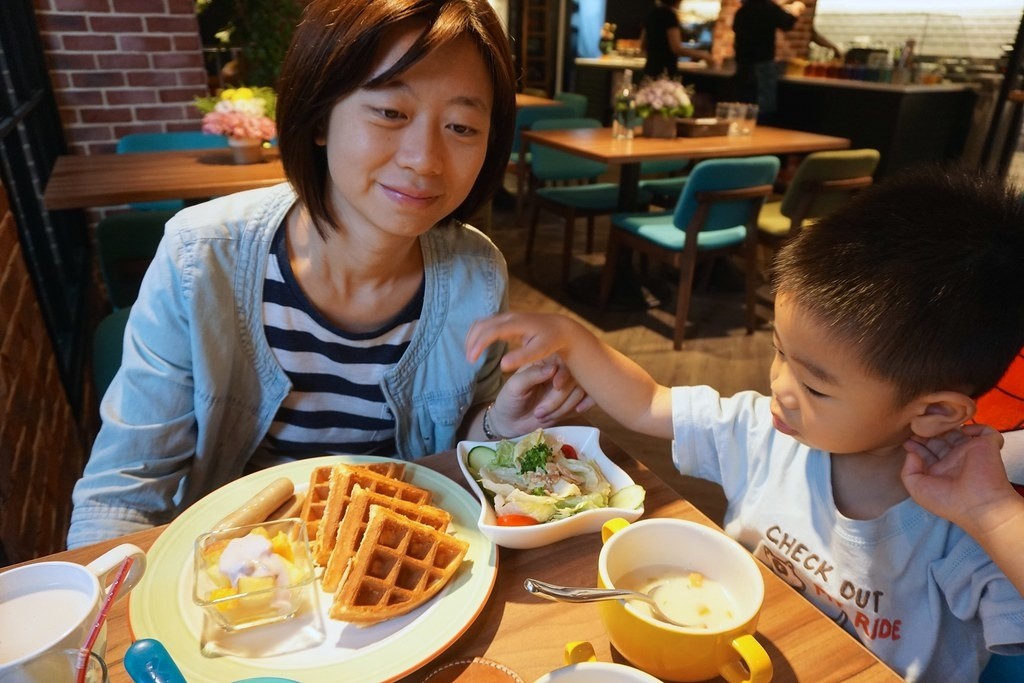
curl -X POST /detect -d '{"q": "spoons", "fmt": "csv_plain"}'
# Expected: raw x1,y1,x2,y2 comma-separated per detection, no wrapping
523,577,705,629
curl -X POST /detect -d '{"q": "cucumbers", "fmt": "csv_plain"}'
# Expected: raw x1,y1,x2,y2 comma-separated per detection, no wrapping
468,445,497,472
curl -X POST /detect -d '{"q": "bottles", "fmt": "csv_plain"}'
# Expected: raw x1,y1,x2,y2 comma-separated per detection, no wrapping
611,68,635,140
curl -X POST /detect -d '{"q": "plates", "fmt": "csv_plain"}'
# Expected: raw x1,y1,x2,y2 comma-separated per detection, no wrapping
127,454,499,683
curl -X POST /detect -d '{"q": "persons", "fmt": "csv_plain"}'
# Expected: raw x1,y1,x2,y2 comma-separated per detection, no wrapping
639,0,714,81
66,0,596,550
466,166,1024,683
812,30,840,58
197,0,308,84
732,0,798,122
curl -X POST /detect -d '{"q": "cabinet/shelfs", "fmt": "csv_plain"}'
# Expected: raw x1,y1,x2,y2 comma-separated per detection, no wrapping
575,57,979,190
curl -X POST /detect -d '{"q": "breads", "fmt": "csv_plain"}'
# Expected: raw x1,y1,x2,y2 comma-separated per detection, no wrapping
293,461,469,621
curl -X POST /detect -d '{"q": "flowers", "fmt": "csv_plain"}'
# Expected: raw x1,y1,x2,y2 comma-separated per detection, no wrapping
201,86,278,141
633,78,695,119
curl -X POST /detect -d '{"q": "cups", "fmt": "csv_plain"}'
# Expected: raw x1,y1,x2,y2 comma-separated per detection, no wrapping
715,101,759,134
0,543,148,683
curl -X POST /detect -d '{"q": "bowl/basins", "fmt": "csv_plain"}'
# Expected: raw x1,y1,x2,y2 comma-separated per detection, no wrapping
191,516,320,634
593,517,774,683
457,423,645,549
530,662,664,683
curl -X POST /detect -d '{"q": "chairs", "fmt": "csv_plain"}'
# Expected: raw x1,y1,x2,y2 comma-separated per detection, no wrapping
91,94,880,412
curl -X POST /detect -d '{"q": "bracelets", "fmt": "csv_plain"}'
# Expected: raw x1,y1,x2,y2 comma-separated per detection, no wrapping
483,402,500,441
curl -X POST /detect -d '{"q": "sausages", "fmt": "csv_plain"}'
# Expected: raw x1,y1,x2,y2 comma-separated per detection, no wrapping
206,477,306,536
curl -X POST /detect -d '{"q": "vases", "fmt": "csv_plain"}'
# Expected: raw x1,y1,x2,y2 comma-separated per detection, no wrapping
643,114,677,139
226,134,272,165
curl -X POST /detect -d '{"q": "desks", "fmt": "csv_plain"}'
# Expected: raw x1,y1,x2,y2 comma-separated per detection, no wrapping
45,153,291,320
527,118,852,309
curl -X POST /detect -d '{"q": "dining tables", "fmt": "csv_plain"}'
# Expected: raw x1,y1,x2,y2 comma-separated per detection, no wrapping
1,416,903,682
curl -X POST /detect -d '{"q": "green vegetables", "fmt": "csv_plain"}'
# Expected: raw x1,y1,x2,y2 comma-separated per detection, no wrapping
516,443,555,475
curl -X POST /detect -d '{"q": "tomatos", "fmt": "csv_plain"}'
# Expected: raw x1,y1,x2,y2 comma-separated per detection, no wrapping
495,514,539,527
